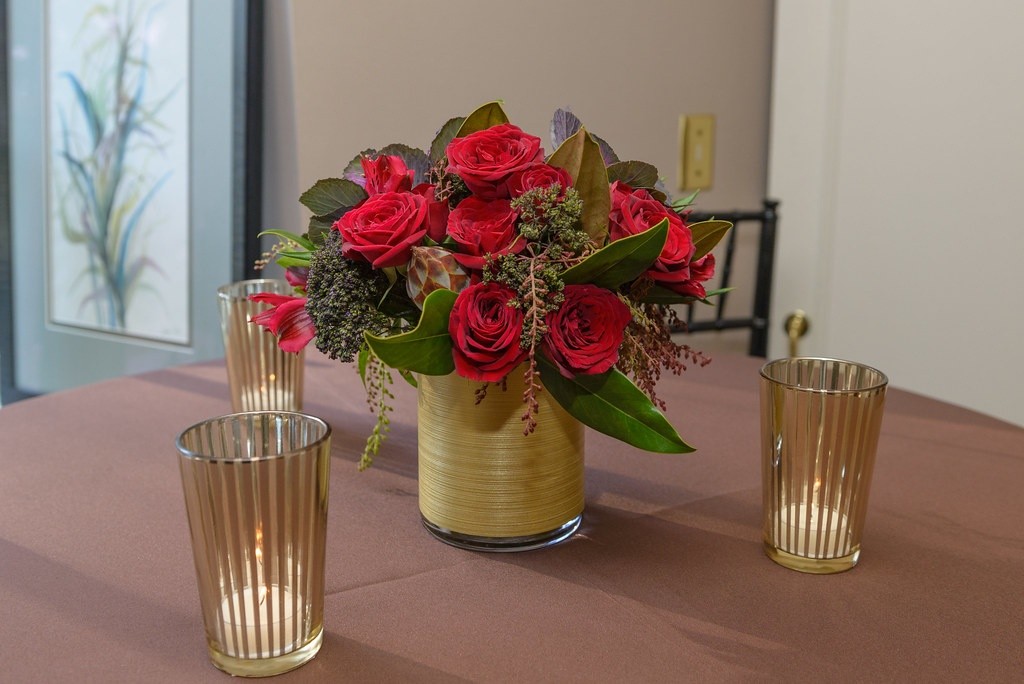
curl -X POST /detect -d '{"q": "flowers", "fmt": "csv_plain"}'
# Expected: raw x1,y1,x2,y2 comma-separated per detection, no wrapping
241,98,740,473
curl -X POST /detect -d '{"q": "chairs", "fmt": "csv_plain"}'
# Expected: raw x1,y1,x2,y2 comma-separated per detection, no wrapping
670,197,781,359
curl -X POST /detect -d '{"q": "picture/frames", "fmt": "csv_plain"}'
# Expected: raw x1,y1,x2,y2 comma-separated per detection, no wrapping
0,0,266,408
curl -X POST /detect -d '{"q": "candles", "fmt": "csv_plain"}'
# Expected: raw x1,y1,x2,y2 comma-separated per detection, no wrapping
769,477,852,559
219,528,303,593
240,374,296,411
212,583,303,659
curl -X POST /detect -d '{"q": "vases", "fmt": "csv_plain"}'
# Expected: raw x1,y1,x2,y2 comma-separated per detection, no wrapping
417,358,587,555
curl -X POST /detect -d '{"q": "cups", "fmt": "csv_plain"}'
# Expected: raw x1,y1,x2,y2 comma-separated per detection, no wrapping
177,411,334,681
761,356,888,572
217,279,307,415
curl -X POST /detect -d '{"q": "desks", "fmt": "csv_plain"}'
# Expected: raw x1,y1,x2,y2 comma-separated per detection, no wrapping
0,343,1024,684
